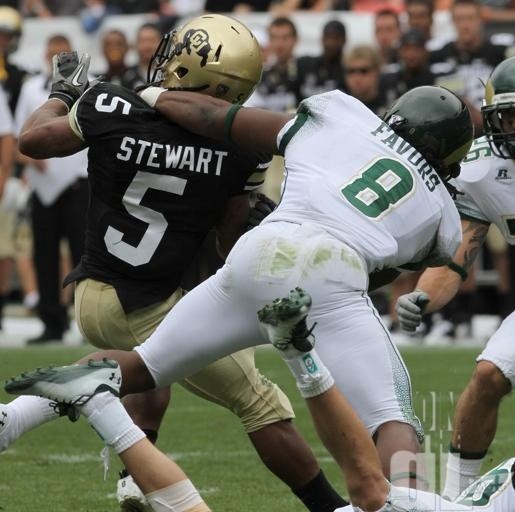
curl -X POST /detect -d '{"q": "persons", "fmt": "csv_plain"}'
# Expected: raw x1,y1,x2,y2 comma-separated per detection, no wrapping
0,1,515,511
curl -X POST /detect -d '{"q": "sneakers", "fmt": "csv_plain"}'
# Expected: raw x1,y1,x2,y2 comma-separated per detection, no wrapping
258,287,317,351
4,359,122,422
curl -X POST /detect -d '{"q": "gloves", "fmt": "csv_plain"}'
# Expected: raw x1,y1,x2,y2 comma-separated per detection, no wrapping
48,50,90,111
396,291,429,331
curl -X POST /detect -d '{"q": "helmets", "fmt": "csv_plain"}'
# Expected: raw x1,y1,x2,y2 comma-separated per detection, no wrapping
382,57,515,182
147,13,263,105
